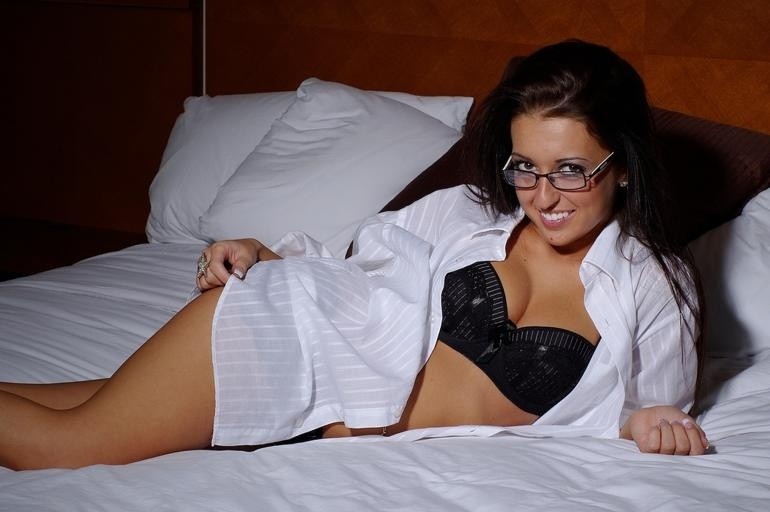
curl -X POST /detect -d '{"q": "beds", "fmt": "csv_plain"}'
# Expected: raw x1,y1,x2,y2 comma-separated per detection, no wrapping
0,104,769,511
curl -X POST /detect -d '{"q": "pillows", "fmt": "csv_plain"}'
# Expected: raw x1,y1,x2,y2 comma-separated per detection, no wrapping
195,75,463,259
145,94,476,245
674,190,768,356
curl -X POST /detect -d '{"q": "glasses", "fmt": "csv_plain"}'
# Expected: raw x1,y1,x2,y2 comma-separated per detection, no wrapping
499,149,618,191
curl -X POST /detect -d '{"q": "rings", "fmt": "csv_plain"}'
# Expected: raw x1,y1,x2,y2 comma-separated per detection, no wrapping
196,250,211,281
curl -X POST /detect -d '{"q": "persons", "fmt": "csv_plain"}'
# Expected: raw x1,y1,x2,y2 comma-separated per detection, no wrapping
0,34,712,471
